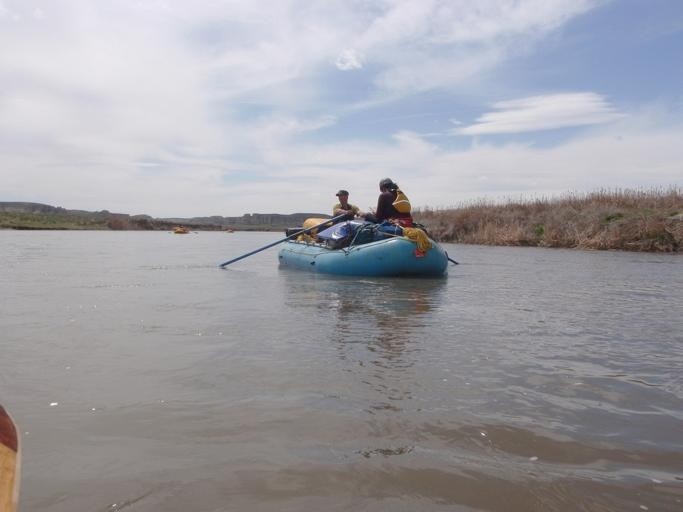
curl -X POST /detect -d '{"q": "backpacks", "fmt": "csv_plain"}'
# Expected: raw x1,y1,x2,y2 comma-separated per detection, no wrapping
391,188,412,213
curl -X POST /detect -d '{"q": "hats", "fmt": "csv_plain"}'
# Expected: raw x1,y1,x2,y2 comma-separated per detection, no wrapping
336,189,349,196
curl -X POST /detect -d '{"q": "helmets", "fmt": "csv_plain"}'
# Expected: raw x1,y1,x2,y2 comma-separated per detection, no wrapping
379,178,393,193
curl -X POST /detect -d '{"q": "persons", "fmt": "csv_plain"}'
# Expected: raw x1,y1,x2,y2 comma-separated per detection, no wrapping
325,289,350,361
356,177,413,226
363,288,427,443
331,189,362,223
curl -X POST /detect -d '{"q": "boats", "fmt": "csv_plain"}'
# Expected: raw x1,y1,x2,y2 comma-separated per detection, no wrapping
174,228,190,235
276,231,455,277
